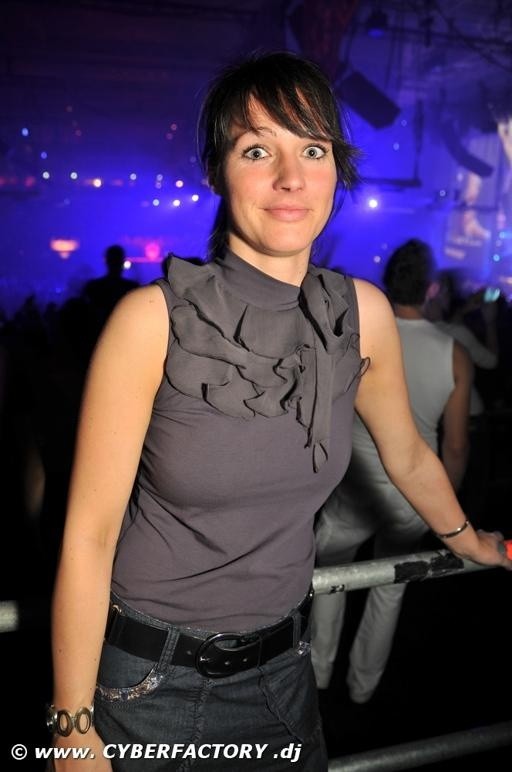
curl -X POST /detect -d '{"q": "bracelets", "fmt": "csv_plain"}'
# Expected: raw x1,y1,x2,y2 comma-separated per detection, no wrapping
44,700,96,738
435,519,470,539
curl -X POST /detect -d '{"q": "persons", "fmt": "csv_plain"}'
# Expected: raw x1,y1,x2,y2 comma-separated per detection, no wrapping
306,237,472,705
46,52,512,772
424,268,512,417
1,244,140,521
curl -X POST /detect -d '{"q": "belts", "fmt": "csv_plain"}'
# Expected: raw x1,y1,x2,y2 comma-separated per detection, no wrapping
105,598,312,679
